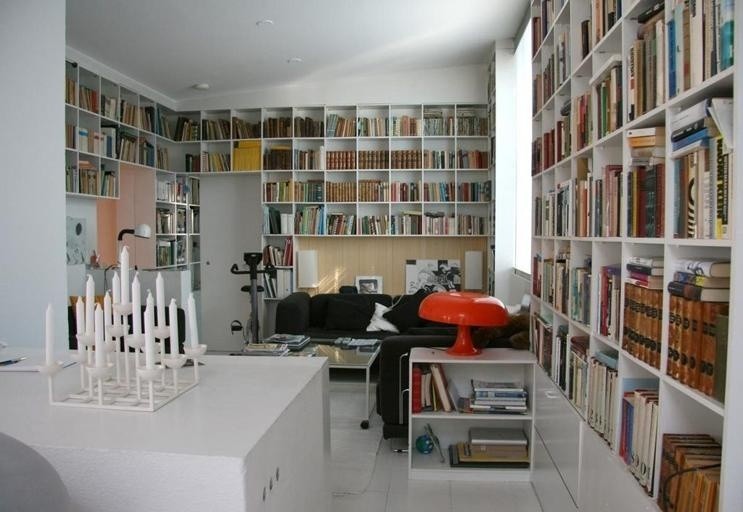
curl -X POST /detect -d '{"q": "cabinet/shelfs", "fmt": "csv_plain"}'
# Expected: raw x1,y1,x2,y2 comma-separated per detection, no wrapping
531,3,683,510
261,171,493,236
406,347,538,482
65,60,156,166
154,105,178,270
64,150,121,198
179,106,492,170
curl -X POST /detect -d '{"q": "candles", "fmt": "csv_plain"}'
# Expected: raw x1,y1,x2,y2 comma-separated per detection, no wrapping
108,271,126,353
184,293,206,384
165,297,188,394
115,244,143,388
126,273,147,401
38,304,65,403
139,289,164,409
100,291,120,371
153,271,172,384
90,303,111,403
71,295,90,389
81,272,99,383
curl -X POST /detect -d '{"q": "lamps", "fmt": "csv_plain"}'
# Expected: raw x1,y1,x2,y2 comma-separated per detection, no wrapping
416,291,513,358
113,221,153,272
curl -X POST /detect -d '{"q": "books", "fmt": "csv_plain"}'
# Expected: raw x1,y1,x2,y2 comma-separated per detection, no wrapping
593,164,623,237
568,334,589,414
101,166,117,196
79,127,99,154
532,97,571,175
594,263,622,342
358,212,390,235
625,127,665,238
669,97,733,242
79,159,99,194
622,255,664,370
458,179,491,202
327,150,356,169
575,53,622,152
570,255,592,328
326,211,357,235
202,118,230,140
264,269,292,299
531,31,569,116
391,210,422,236
326,180,356,203
553,324,568,392
581,0,621,61
457,145,488,169
424,211,455,236
457,115,488,137
66,78,75,106
66,165,76,192
66,121,77,150
294,206,323,235
358,116,388,136
587,351,618,451
295,116,324,137
101,94,118,121
157,142,169,171
263,116,291,137
232,116,261,139
326,114,356,137
263,237,292,267
626,0,734,121
391,115,422,137
294,145,325,171
202,151,230,172
359,180,388,203
412,363,530,415
262,178,293,202
534,159,592,238
391,180,421,202
156,207,174,234
656,431,720,512
233,140,261,170
79,85,98,113
533,244,570,316
184,153,200,173
242,333,310,357
100,124,137,163
263,145,291,169
457,213,488,235
359,150,389,170
173,116,200,141
530,310,553,377
424,181,455,202
121,99,137,126
619,388,658,496
294,180,324,203
157,106,171,139
139,136,155,166
424,149,456,170
448,426,530,468
156,238,186,266
391,149,421,169
424,110,454,136
263,206,293,235
158,176,200,204
139,105,155,132
177,207,200,234
0,357,77,373
533,0,567,55
665,255,730,408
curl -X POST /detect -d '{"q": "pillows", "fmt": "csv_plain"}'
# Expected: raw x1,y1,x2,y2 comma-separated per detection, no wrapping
368,302,402,335
323,296,367,334
385,287,433,329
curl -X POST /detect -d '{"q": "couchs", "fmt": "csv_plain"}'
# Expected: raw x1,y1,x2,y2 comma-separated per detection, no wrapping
276,293,528,454
